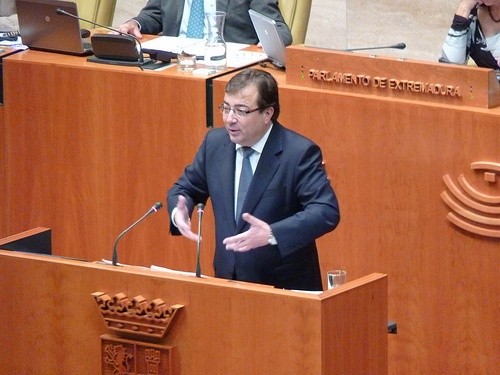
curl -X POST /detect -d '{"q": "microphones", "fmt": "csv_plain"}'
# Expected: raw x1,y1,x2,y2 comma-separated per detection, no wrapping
194,203,204,277
55,8,152,66
347,42,406,52
112,201,163,266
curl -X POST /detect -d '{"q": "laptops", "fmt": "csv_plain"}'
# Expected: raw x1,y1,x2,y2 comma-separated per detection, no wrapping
15,0,96,56
247,8,288,70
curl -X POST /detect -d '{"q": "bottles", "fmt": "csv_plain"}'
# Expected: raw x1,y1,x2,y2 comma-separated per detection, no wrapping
203,11,228,72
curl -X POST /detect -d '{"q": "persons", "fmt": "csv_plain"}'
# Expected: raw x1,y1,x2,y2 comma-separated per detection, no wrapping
107,0,293,50
439,0,500,69
164,70,340,291
0,0,20,33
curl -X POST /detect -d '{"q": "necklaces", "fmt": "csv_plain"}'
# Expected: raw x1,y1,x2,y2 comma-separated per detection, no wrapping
487,6,500,23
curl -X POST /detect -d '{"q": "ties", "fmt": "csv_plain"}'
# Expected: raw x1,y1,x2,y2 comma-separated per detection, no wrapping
186,0,204,38
236,146,255,225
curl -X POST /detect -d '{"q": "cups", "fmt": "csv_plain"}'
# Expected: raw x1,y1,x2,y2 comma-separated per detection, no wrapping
327,270,347,290
177,48,196,72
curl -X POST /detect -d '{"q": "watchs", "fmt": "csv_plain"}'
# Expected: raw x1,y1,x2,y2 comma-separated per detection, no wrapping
267,231,278,246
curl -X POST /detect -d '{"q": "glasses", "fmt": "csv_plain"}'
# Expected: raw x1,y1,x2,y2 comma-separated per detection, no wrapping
217,103,273,118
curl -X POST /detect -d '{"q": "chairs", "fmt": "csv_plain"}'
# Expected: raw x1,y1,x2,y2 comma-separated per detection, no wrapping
65,0,116,39
278,0,312,46
